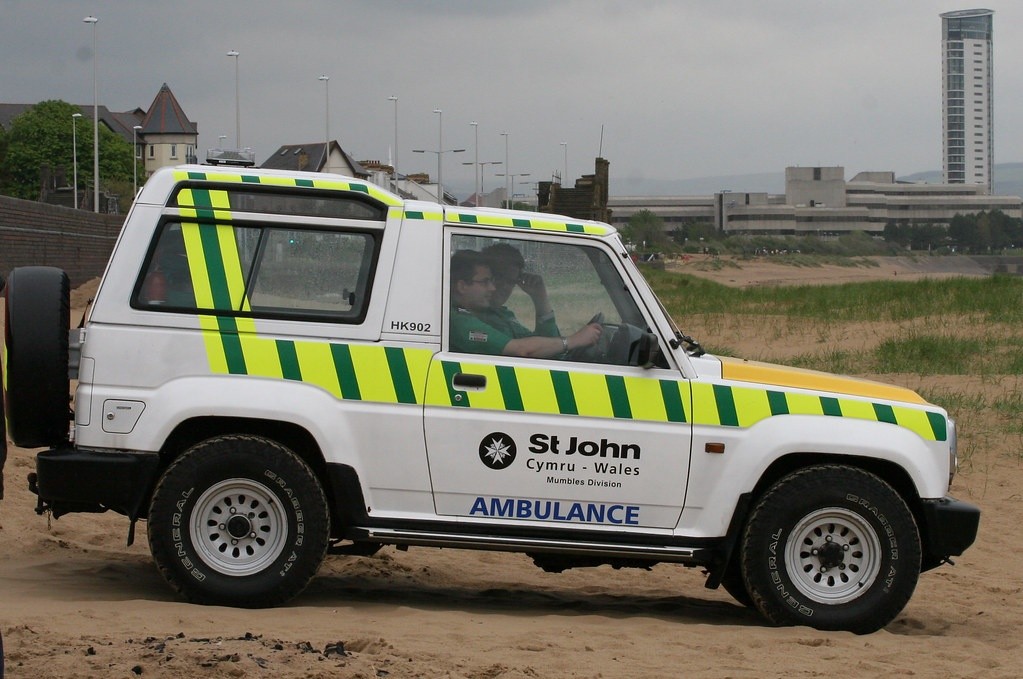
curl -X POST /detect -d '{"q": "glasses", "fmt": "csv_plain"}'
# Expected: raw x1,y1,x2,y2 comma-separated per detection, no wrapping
499,275,517,287
467,275,495,286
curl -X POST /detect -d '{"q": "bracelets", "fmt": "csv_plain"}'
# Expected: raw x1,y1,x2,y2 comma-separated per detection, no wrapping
561,337,569,353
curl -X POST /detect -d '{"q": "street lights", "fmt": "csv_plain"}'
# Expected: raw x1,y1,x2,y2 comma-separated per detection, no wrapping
501,133,509,209
470,120,479,207
463,162,504,207
560,142,568,188
133,126,143,200
413,149,466,204
218,135,227,152
83,15,99,214
519,181,539,212
318,74,331,173
387,95,399,194
496,173,530,210
72,113,82,209
433,107,443,204
226,50,241,153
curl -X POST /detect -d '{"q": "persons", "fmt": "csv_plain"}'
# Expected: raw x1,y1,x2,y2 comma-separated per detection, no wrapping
481,242,561,338
447,248,604,359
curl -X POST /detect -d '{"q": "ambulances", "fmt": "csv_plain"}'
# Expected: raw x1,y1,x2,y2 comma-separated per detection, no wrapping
2,163,984,636
